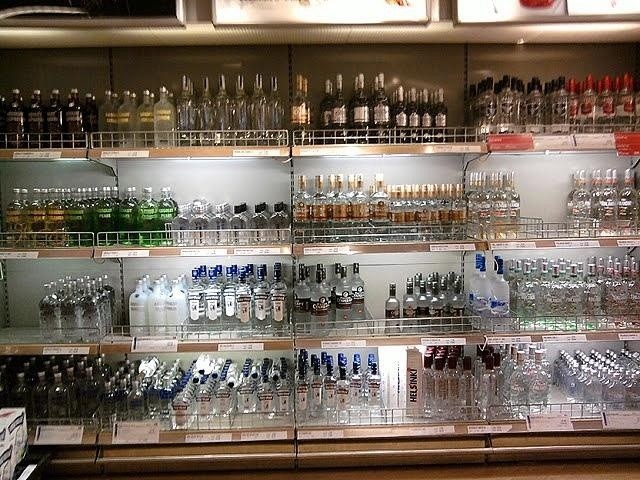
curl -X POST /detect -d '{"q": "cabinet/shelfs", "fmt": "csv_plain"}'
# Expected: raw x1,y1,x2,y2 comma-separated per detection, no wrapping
291,126,491,467
0,128,115,478
87,125,298,473
485,118,640,462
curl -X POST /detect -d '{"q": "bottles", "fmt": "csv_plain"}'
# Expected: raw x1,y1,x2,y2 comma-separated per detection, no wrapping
553,349,640,414
471,254,639,333
131,262,288,335
294,173,520,242
385,272,464,337
422,347,551,418
321,74,448,144
1,91,99,149
467,73,640,146
565,169,640,231
100,85,175,148
0,349,380,425
38,273,116,341
294,262,365,335
4,189,292,249
177,73,284,146
292,74,311,143
